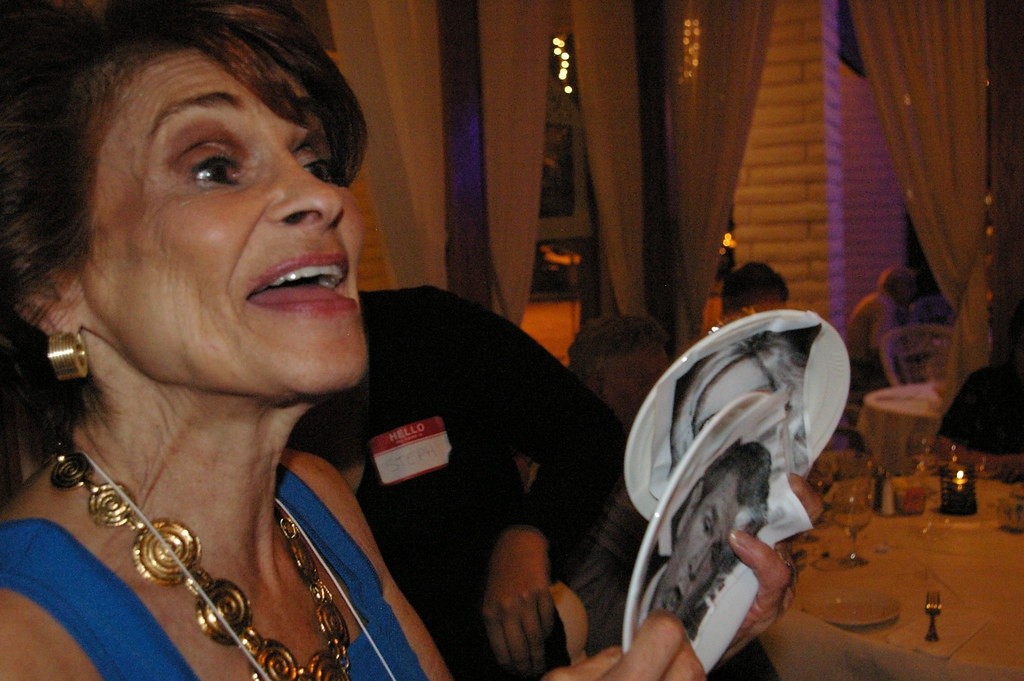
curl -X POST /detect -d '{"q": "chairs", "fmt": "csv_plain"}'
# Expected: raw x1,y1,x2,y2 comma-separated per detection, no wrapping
881,323,956,388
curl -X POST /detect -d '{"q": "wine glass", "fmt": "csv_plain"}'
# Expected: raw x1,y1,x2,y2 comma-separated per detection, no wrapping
830,486,873,567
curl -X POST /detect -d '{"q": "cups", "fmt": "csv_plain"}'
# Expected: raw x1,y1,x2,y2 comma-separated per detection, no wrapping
892,475,926,515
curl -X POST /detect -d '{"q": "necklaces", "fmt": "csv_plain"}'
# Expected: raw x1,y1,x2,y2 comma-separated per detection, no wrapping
49,440,356,681
74,452,397,681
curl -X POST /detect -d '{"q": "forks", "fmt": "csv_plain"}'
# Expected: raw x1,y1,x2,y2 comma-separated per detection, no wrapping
925,591,942,641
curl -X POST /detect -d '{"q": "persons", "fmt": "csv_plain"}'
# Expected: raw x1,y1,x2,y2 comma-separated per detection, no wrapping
669,331,811,488
569,309,670,437
844,263,929,390
638,436,775,640
925,302,1024,488
0,0,825,680
283,286,784,681
715,260,791,334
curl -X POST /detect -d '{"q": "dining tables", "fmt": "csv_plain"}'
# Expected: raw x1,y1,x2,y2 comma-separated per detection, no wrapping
754,470,1024,681
853,382,948,472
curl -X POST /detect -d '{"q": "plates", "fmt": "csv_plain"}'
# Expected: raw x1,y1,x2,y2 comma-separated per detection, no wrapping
624,309,852,521
622,390,790,677
805,594,901,628
